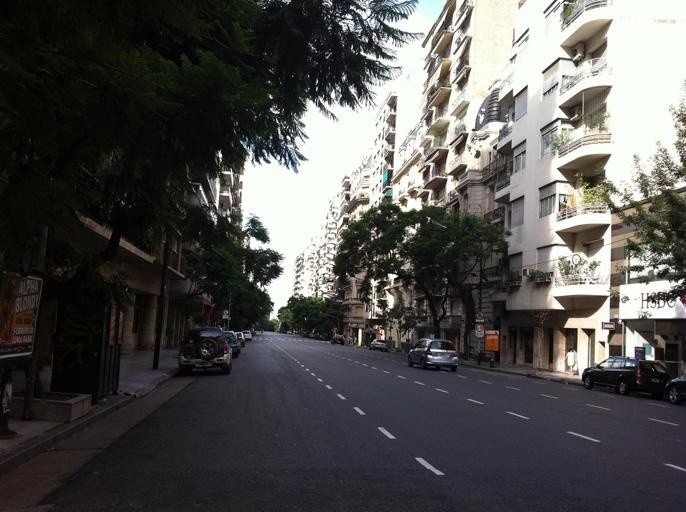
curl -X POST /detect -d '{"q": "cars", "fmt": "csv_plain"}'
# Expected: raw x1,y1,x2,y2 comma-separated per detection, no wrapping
285,327,345,346
368,339,389,353
664,374,686,405
223,327,256,357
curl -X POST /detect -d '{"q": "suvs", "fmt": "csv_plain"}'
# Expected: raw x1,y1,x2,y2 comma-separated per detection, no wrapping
580,354,669,397
174,326,233,376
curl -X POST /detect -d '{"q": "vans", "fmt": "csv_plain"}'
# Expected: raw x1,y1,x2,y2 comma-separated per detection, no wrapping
405,337,458,372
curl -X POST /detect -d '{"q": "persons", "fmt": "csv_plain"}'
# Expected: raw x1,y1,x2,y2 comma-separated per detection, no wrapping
350,337,357,345
563,348,575,372
